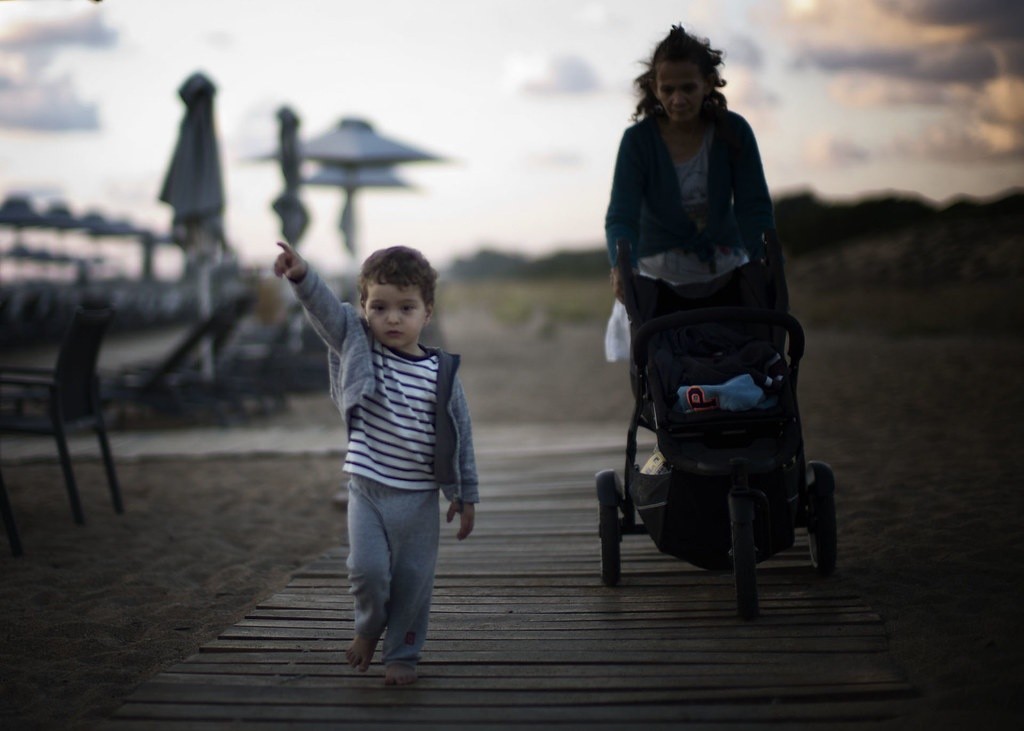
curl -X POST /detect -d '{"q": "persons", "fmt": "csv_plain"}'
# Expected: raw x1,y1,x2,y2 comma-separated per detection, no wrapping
275,240,482,691
606,26,785,427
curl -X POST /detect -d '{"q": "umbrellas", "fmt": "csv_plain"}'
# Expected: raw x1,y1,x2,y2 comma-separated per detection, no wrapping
275,105,320,299
256,121,439,254
160,74,225,384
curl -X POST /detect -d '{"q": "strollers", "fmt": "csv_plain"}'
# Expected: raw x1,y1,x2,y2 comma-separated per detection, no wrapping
594,232,840,622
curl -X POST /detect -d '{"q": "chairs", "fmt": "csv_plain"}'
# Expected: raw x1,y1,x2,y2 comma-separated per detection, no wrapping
0,299,120,556
